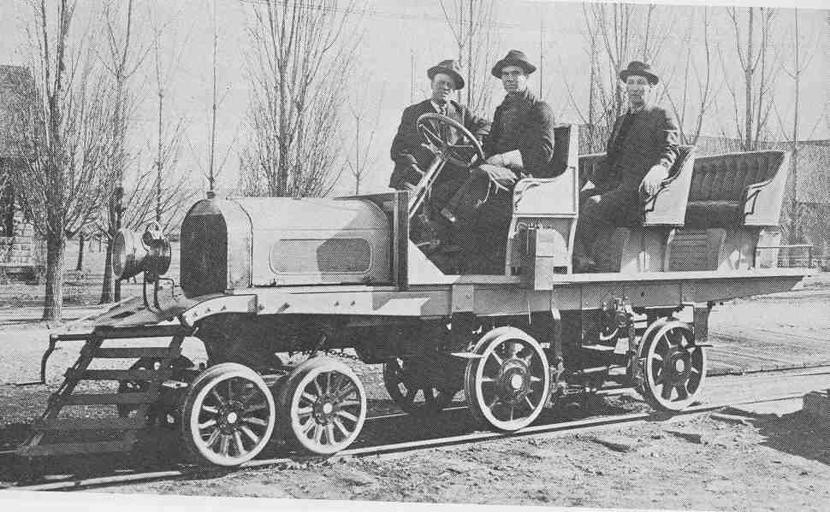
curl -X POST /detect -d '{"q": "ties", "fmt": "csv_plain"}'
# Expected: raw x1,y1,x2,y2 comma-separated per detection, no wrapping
439,105,446,140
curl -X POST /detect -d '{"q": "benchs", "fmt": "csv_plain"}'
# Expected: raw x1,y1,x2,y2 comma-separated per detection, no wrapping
581,145,698,272
672,150,790,270
471,121,582,272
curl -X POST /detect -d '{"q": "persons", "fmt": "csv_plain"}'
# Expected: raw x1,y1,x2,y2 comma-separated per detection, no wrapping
577,59,680,273
387,60,494,202
402,49,556,257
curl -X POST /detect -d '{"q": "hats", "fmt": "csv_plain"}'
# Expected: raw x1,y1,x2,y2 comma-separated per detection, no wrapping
426,59,465,90
489,48,537,79
619,60,660,86
